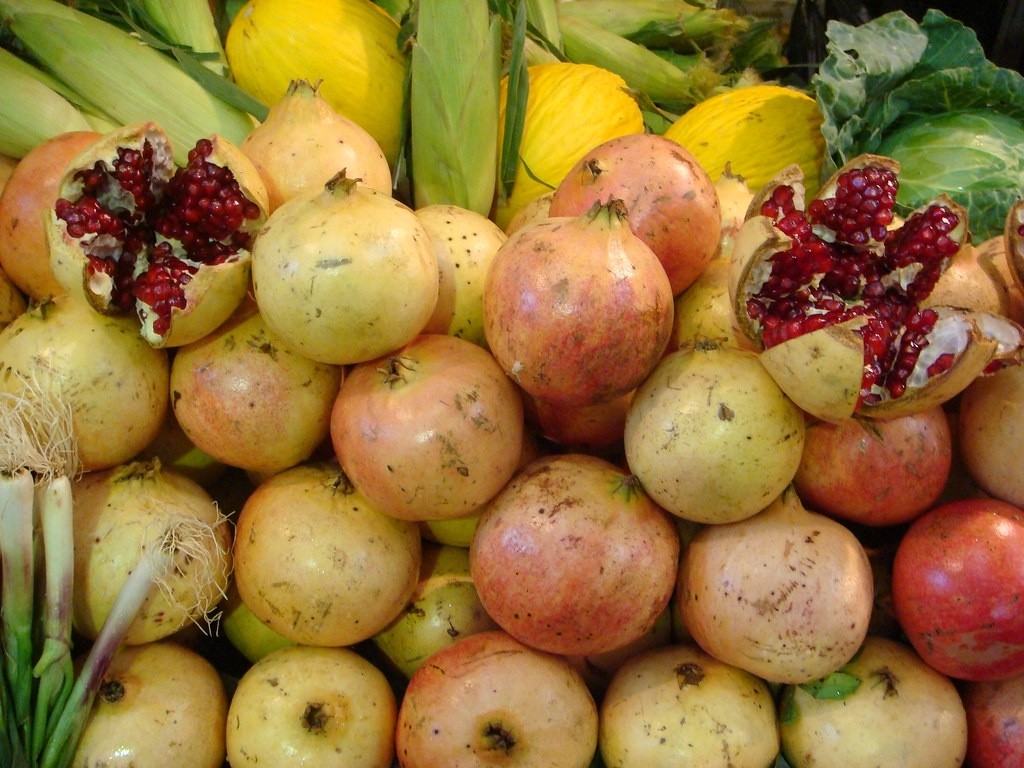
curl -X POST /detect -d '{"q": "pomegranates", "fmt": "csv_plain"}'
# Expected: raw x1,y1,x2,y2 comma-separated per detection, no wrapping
0,76,1024,767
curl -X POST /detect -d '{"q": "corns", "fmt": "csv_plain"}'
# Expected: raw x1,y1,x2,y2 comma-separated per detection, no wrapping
0,0,789,222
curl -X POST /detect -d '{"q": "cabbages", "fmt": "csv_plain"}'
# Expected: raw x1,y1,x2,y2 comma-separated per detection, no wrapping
808,7,1024,247
